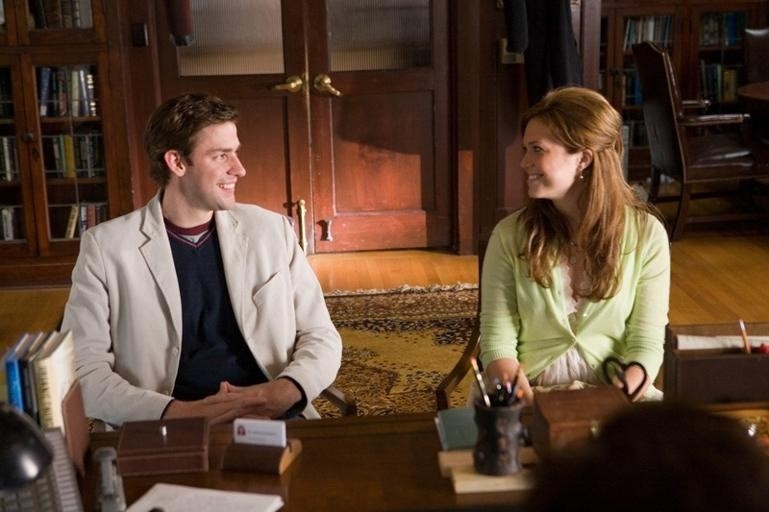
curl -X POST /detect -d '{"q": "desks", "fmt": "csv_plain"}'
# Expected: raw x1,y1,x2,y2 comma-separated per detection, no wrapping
74,399,768,511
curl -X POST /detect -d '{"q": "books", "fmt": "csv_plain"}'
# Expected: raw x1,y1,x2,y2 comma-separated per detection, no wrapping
433,404,481,450
623,12,738,108
122,481,285,512
0,0,110,241
3,330,74,437
452,467,536,503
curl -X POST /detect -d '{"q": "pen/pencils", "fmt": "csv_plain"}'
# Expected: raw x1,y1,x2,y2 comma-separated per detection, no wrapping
736,316,769,355
470,356,524,407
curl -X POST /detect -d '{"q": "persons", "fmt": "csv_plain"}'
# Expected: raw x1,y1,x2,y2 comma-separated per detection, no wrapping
469,85,673,402
58,90,344,425
522,398,769,512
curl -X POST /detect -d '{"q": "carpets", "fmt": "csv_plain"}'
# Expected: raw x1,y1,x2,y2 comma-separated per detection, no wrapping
307,282,484,419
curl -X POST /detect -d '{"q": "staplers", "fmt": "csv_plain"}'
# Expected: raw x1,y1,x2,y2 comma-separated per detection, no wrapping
92,446,127,512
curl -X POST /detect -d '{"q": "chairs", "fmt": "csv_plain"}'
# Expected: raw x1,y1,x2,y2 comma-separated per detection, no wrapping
630,39,768,240
436,237,494,410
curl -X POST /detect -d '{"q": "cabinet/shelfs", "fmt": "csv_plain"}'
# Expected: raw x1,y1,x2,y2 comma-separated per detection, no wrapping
599,0,769,222
0,0,143,287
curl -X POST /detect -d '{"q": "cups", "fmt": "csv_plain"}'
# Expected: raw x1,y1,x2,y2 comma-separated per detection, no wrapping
473,392,526,476
